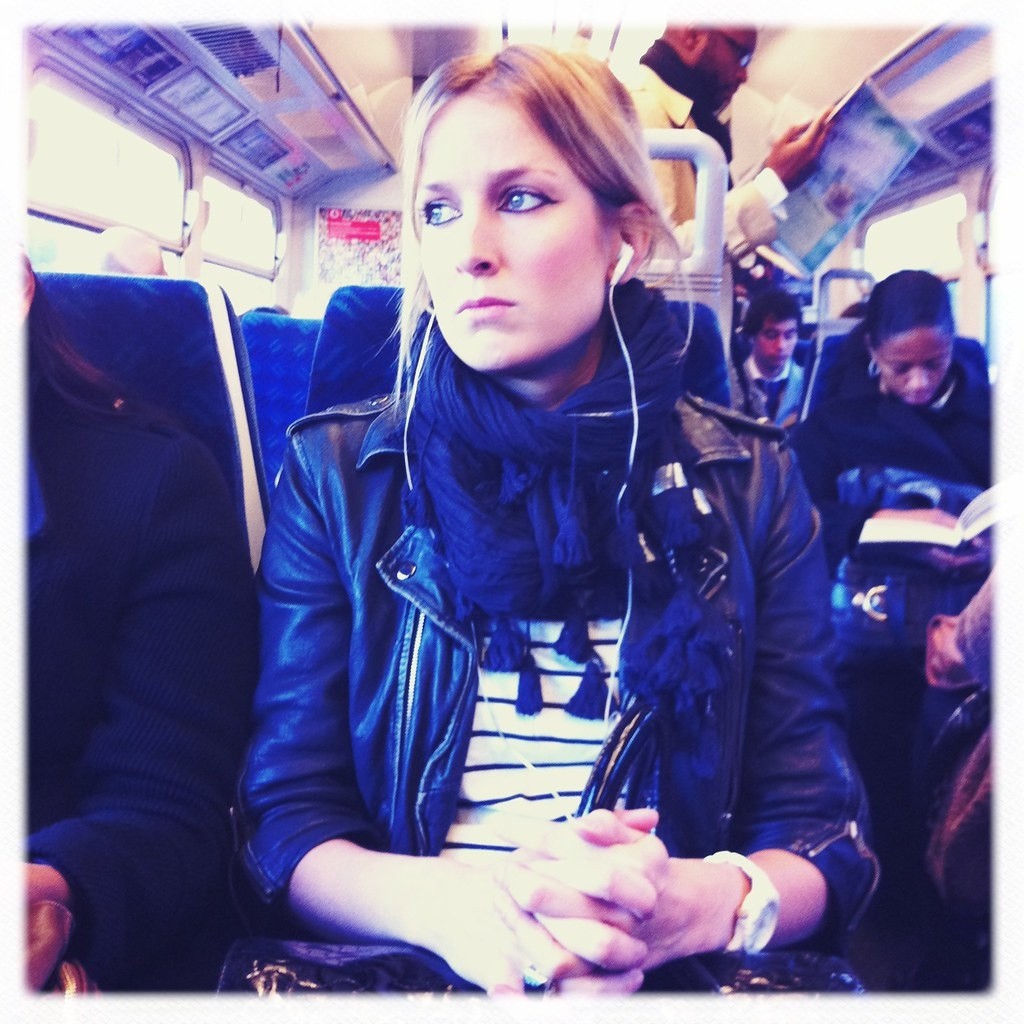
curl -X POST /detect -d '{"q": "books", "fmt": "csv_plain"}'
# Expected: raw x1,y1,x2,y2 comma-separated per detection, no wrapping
859,483,991,548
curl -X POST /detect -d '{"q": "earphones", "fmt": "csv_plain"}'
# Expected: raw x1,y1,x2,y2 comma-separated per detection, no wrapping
610,240,635,286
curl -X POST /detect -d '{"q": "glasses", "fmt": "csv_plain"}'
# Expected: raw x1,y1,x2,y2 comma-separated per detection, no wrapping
717,30,753,68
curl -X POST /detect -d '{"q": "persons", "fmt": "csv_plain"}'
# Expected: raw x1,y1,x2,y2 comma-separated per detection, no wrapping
789,270,991,937
623,19,831,364
224,42,881,996
25,254,257,994
741,287,803,432
829,465,990,992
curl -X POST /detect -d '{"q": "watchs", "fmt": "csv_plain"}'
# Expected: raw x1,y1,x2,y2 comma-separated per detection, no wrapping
706,849,780,958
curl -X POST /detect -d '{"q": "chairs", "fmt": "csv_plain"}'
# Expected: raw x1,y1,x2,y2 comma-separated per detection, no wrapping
29,129,994,998
